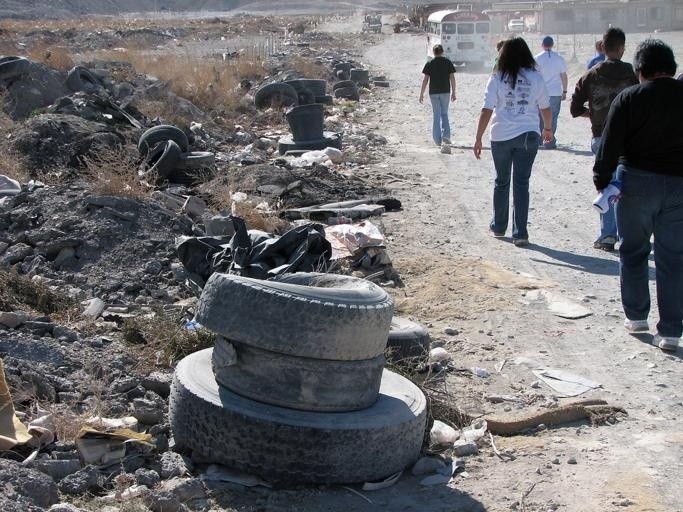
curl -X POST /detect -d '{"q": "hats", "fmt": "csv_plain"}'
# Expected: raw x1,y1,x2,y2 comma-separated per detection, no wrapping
543,36,554,46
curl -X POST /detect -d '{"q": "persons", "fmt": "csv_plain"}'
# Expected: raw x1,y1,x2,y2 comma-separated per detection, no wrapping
586,40,605,69
591,36,682,353
491,40,506,71
417,44,457,146
531,36,568,149
569,27,640,253
472,36,554,247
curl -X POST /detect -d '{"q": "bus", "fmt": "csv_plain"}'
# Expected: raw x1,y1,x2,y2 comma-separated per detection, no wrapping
425,9,492,68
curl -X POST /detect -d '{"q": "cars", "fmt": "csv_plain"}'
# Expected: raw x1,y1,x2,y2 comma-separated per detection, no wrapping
504,19,526,32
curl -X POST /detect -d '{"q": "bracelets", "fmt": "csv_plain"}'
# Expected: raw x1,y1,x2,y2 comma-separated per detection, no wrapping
562,91,567,93
543,128,552,131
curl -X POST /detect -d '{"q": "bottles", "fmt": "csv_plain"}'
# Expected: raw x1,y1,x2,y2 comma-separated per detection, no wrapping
592,180,624,216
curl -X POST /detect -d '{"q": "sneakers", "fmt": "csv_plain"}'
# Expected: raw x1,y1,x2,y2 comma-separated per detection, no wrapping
593,239,616,252
435,137,452,146
624,317,679,351
488,219,529,247
